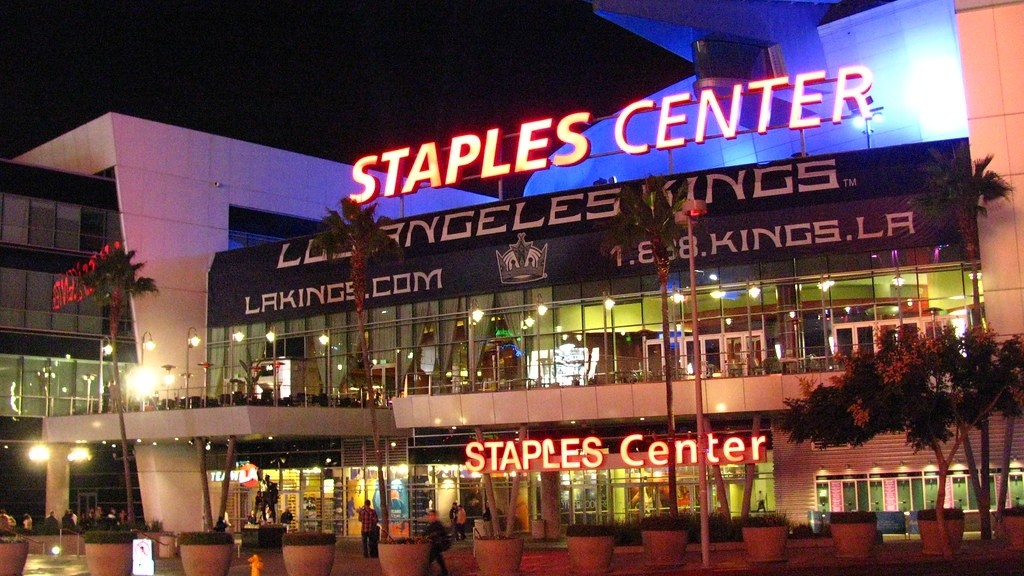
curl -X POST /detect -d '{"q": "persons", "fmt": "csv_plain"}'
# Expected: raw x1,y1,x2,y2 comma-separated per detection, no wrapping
102,379,116,413
449,502,466,541
420,509,448,576
756,490,766,512
23,514,32,531
62,509,78,528
483,502,491,537
0,508,16,530
216,516,227,532
257,471,278,523
45,510,58,528
358,499,379,558
247,491,292,532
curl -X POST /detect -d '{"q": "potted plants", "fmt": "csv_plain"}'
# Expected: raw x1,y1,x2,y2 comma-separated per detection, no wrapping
1001,504,1024,551
179,532,234,576
917,508,965,555
0,537,29,576
282,532,336,576
741,508,790,562
567,525,616,576
378,538,433,576
829,511,878,558
639,514,690,570
83,531,138,576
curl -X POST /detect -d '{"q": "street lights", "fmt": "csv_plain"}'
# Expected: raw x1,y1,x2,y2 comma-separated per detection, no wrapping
674,199,711,567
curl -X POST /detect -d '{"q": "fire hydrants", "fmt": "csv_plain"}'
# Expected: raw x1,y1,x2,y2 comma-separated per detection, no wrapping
247,554,264,576
